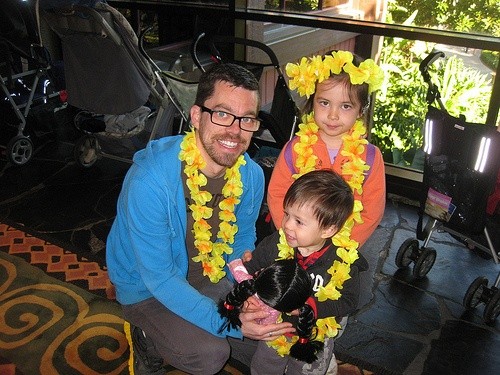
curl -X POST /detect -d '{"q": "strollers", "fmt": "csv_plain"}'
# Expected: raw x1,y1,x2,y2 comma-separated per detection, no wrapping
395,52,500,326
137,23,207,132
0,0,71,167
211,35,305,157
40,0,196,174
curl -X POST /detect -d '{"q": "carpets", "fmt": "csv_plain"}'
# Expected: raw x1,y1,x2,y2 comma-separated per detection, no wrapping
0,220,252,375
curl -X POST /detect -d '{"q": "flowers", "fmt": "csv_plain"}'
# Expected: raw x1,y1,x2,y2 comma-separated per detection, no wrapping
179,126,247,284
285,50,385,100
292,109,369,238
266,228,360,358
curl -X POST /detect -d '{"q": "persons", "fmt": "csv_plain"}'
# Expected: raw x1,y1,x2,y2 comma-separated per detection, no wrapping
106,62,257,375
267,49,385,250
240,167,369,375
217,257,325,364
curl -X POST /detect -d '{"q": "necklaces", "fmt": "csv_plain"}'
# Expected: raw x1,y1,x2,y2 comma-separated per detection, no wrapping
274,225,360,338
289,106,370,238
177,126,249,284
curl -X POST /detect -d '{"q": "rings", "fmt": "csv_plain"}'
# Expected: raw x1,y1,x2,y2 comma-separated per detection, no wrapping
269,332,272,338
